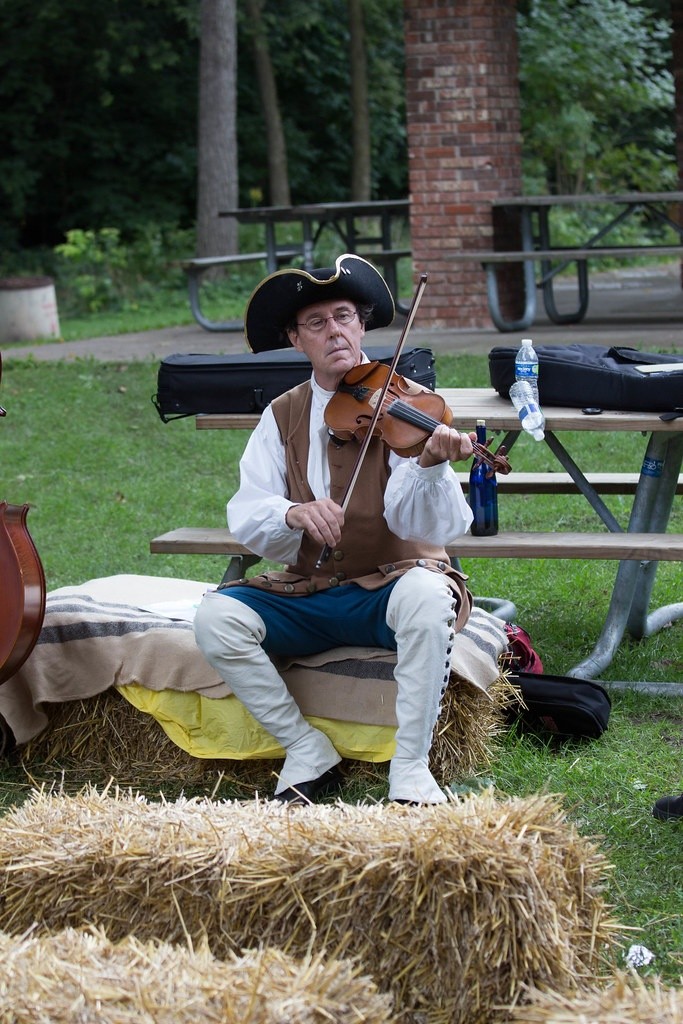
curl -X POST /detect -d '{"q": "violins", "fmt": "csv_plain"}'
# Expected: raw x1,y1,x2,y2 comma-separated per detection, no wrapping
322,359,516,477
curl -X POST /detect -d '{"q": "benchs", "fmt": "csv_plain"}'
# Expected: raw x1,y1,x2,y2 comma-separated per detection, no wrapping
455,470,683,497
182,249,302,323
357,250,412,303
443,242,683,322
147,523,683,584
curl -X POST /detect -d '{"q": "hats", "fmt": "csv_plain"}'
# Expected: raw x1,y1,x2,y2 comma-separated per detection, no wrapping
244,254,397,355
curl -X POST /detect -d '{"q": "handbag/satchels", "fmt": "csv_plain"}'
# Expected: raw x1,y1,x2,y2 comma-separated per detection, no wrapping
505,622,543,674
489,344,683,421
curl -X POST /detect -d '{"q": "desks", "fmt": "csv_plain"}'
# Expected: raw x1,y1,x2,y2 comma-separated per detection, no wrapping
193,386,683,692
181,199,413,331
443,195,683,332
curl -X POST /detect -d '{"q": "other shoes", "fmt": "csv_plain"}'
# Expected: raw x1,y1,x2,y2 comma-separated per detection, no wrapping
282,762,343,804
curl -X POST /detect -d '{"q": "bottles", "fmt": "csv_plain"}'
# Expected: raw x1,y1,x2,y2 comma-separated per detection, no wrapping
509,380,545,441
515,339,539,407
469,419,499,536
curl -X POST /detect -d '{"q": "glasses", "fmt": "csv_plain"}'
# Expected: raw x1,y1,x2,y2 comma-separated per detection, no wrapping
292,310,359,332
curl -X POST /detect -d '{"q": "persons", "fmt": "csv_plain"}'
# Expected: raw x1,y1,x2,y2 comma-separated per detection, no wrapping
194,253,478,805
